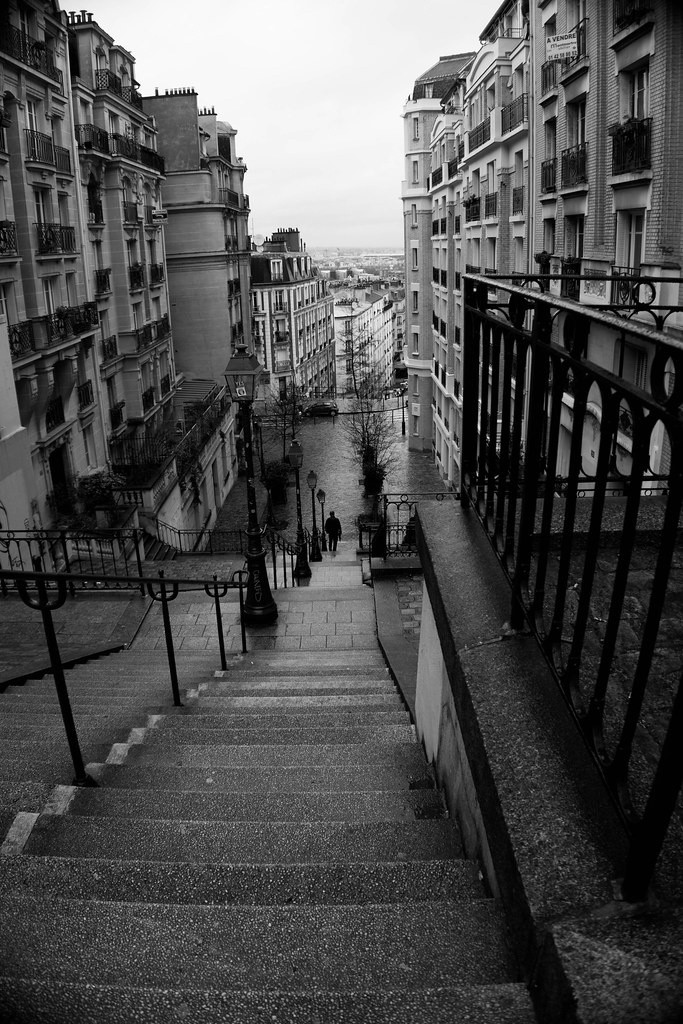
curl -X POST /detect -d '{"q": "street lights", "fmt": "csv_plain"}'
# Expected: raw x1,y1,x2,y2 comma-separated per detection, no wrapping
399,384,407,434
306,469,322,563
288,438,313,586
221,343,280,628
316,488,327,552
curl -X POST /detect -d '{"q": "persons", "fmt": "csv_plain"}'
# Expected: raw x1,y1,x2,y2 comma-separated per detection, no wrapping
325,511,342,552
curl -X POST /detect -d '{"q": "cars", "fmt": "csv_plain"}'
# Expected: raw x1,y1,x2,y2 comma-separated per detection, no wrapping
304,401,339,418
